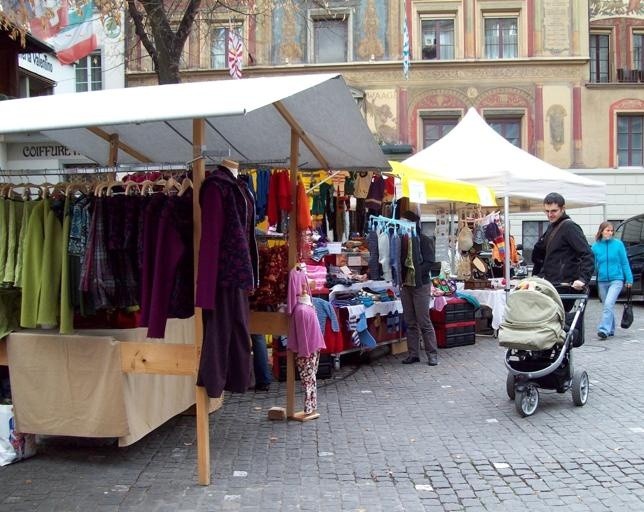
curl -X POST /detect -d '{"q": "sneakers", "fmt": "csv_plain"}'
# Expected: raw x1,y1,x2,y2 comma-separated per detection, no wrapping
597,331,608,340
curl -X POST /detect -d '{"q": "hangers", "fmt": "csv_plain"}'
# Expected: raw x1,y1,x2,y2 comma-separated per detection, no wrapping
1,161,193,198
239,157,287,174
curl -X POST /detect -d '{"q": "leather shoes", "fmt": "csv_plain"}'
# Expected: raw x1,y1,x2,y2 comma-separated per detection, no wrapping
401,356,422,364
428,357,438,366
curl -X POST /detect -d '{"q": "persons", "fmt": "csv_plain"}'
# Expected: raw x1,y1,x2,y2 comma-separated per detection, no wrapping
287,287,327,417
397,210,438,366
193,154,259,399
530,225,550,274
533,191,594,305
589,220,633,339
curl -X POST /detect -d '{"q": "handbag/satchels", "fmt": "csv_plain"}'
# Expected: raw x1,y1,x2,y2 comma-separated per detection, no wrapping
617,283,635,330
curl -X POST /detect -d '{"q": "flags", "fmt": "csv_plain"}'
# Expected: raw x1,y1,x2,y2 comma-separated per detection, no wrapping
224,20,245,79
402,3,412,80
27,1,98,66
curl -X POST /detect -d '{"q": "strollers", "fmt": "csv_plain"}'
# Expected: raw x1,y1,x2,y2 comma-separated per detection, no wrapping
497,277,589,417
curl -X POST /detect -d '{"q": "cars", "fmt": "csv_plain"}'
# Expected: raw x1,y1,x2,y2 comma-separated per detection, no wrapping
589,213,644,302
